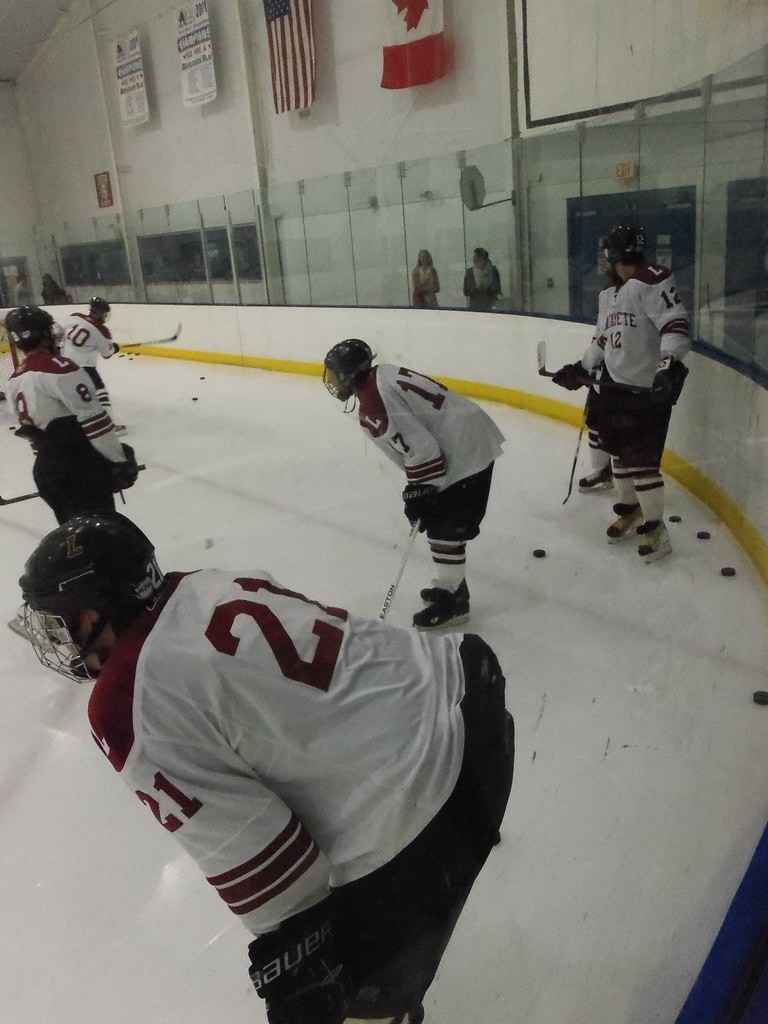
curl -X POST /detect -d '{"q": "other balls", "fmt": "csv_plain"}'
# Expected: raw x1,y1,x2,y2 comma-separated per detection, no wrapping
119,355,124,357
135,353,139,355
697,531,710,539
200,377,206,380
533,549,546,557
129,358,133,360
753,690,768,705
193,398,198,400
669,516,681,522
9,426,16,430
127,353,131,354
721,567,735,576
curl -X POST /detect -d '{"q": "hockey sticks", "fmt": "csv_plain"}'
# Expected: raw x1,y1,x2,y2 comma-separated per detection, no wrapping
120,322,182,349
536,340,652,396
377,516,422,624
0,464,148,506
561,370,596,506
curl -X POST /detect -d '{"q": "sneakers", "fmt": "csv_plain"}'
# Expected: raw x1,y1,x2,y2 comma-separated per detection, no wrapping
420,578,441,608
578,457,614,492
634,518,673,564
413,581,470,632
607,503,644,545
115,425,128,437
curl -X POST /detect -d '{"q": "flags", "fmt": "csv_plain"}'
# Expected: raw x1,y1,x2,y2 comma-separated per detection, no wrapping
263,0,316,114
381,0,447,89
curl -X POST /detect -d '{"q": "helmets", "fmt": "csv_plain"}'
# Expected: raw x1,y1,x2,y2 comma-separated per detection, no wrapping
90,296,110,323
598,222,647,264
18,513,165,687
7,305,53,351
322,339,372,403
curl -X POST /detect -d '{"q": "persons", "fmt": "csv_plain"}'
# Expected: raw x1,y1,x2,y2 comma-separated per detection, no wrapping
554,224,691,563
11,512,515,1024
324,339,505,632
7,306,137,524
15,275,31,306
53,297,127,437
41,273,66,305
463,247,501,309
411,250,439,307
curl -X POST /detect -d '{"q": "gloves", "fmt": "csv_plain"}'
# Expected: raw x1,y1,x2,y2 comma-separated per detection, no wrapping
554,361,589,390
112,343,119,354
245,895,361,1024
402,484,439,533
652,355,689,406
103,442,139,494
